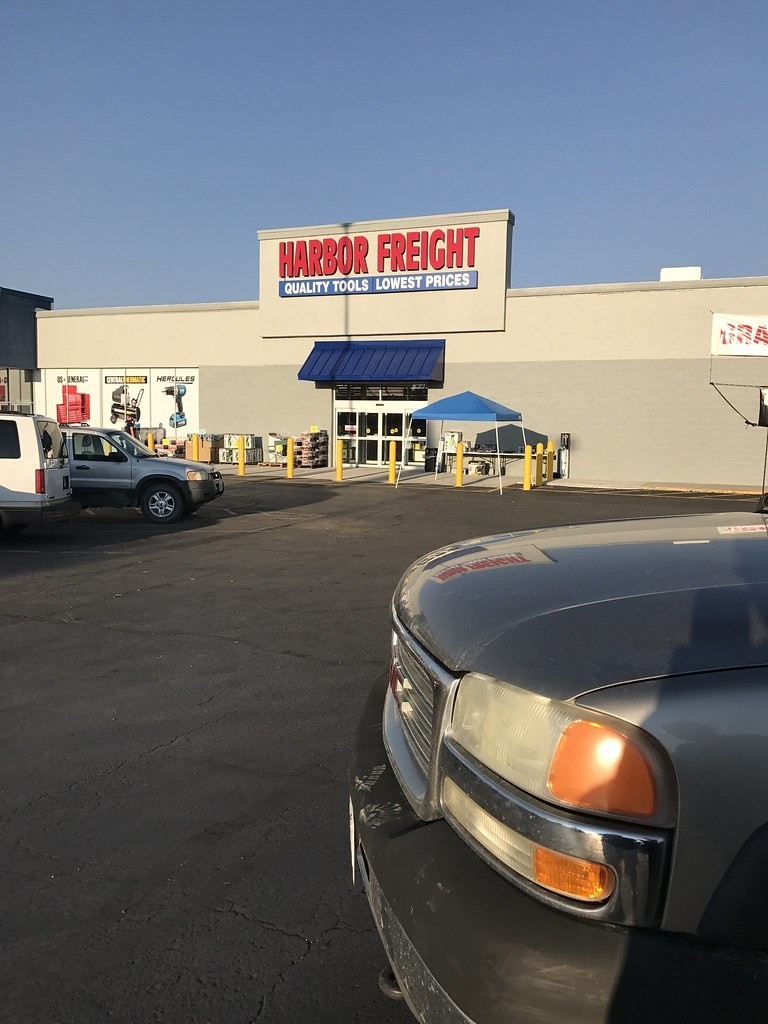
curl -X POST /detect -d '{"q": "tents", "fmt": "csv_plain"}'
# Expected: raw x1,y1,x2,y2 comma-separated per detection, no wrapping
395,391,533,495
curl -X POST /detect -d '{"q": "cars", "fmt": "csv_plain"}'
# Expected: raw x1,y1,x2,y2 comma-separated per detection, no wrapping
58,423,225,524
344,506,768,1024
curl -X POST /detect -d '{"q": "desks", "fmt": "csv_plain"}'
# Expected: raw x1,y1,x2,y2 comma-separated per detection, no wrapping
435,451,556,481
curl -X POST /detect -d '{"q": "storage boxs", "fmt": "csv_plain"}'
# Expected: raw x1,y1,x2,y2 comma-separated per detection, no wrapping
154,430,328,469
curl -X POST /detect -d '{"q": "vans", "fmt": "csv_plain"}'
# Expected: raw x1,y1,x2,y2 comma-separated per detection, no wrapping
0,410,82,533
